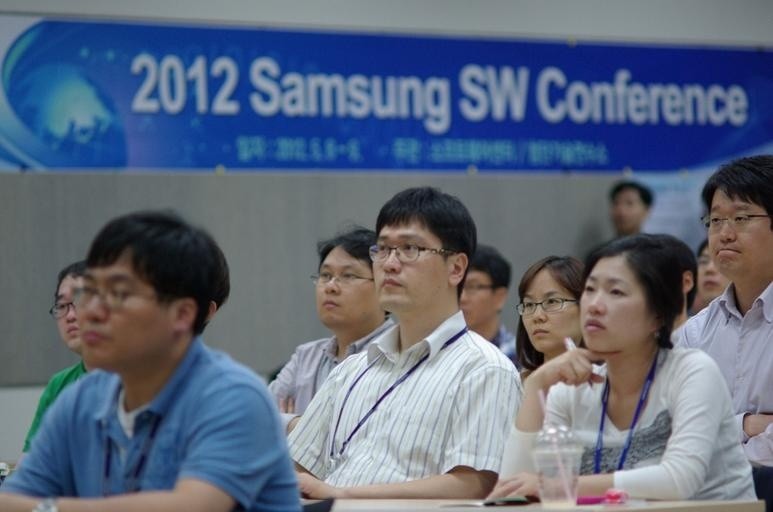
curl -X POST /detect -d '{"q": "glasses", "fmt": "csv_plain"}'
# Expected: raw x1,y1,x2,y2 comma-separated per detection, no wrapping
369,243,456,265
515,296,578,316
71,287,156,312
700,213,768,230
310,271,374,289
50,300,80,319
697,254,714,268
462,281,492,293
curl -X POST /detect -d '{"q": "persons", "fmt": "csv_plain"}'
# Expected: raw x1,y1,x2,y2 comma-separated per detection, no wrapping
516,256,585,388
458,244,519,369
670,154,773,510
285,186,522,501
201,251,231,335
653,234,730,321
0,210,306,512
22,257,93,464
607,181,653,237
486,232,760,502
267,221,393,439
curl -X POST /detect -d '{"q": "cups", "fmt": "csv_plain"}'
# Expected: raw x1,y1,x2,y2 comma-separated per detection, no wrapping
532,443,585,507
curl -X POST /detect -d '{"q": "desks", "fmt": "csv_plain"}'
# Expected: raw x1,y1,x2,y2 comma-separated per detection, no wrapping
331,496,767,512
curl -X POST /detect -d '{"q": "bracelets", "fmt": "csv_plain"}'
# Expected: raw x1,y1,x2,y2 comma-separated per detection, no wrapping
33,496,56,512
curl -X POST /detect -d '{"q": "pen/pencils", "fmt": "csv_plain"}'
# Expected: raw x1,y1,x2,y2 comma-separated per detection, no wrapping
563,336,592,387
